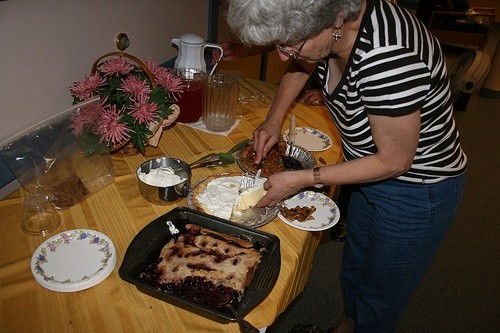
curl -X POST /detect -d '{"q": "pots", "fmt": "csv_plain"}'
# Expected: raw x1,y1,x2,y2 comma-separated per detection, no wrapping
119,207,282,324
136,153,220,206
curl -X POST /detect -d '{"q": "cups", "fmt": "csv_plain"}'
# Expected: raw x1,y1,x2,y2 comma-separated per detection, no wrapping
202,74,239,131
21,195,61,235
170,68,207,124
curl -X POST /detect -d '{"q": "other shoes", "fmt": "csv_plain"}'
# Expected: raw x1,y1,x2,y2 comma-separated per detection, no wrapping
289,323,335,333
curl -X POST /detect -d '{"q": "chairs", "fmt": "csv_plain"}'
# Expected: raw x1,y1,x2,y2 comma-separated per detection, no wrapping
427,7,496,112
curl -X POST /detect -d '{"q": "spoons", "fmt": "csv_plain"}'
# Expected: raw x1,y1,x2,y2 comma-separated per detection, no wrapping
216,295,260,333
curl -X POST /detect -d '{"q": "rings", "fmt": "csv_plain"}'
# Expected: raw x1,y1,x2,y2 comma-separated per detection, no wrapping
315,100,320,105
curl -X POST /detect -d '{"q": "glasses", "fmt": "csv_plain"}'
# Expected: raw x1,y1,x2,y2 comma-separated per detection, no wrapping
275,37,309,61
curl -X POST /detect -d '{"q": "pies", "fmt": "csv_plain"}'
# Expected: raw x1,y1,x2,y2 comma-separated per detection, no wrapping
191,172,268,224
239,140,295,178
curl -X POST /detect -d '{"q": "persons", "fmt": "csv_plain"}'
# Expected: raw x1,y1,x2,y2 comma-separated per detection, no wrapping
226,0,469,333
212,38,353,242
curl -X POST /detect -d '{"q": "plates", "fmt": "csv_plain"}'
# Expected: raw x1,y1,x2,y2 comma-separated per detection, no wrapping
31,228,118,292
236,141,316,177
187,172,280,228
276,191,340,231
282,127,333,152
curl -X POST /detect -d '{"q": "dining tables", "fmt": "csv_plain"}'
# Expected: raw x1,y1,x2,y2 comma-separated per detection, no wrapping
0,75,343,333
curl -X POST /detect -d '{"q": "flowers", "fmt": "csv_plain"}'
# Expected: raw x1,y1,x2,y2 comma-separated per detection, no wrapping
67,57,186,160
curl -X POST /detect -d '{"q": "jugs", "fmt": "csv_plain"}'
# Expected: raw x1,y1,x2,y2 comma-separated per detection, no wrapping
172,34,224,76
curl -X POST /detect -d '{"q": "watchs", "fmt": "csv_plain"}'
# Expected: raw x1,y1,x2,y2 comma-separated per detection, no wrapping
313,166,325,189
